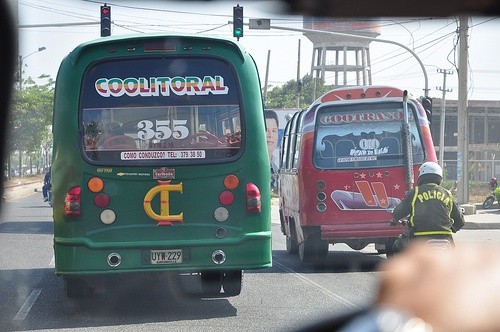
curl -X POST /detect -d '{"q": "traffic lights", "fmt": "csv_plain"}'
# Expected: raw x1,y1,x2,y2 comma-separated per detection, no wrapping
421,98,433,121
100,6,111,37
233,6,244,38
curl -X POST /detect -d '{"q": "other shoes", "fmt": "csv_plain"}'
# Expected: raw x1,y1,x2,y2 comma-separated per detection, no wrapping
43,197,48,202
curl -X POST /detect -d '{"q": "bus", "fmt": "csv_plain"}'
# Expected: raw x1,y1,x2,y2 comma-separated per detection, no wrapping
275,85,438,268
48,33,274,295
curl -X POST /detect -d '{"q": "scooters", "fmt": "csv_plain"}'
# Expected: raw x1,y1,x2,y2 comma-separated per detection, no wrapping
390,217,457,258
482,177,498,209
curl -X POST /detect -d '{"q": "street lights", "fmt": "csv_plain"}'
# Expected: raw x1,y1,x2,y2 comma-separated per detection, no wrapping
19,47,47,91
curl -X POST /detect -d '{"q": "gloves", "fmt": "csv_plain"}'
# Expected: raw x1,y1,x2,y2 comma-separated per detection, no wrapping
390,218,399,226
451,223,459,233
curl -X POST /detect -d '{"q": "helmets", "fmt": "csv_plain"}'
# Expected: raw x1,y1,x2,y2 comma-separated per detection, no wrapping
490,177,497,183
417,161,443,179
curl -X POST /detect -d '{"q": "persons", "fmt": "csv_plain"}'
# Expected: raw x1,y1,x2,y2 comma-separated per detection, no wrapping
104,122,124,149
489,177,497,201
391,162,465,255
43,170,51,202
340,239,500,332
265,110,280,195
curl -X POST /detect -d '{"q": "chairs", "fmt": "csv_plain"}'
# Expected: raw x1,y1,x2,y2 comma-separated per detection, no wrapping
315,136,422,168
83,129,241,150
